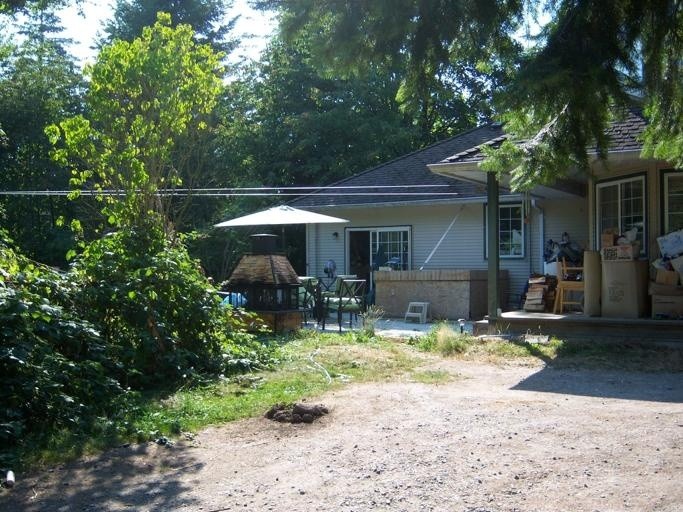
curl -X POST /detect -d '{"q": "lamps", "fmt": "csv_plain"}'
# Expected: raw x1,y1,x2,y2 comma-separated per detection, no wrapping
332,232,338,240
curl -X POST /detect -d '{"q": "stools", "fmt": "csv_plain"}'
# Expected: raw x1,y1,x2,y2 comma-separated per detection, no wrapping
404,301,432,323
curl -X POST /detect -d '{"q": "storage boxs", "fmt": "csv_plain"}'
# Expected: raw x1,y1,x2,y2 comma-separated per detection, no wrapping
601,228,683,320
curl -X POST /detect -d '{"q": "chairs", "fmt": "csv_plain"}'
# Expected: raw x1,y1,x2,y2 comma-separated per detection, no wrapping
550,256,585,315
291,274,367,333
501,288,522,312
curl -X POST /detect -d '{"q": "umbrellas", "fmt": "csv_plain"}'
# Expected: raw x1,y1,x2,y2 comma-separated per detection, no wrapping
212,204,351,257
